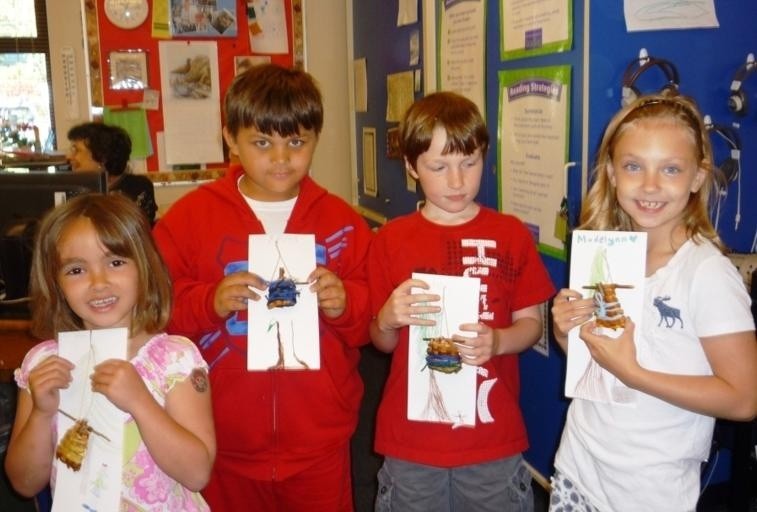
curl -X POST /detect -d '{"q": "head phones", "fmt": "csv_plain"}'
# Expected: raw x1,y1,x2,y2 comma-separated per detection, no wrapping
620,57,680,108
706,124,740,187
726,62,756,116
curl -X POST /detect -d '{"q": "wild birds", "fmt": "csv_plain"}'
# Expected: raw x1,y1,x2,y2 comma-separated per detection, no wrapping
169,58,191,76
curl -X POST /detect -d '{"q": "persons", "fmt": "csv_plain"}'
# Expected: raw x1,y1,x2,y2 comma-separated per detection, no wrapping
539,91,757,511
2,192,221,512
352,90,557,510
145,64,381,511
61,122,157,229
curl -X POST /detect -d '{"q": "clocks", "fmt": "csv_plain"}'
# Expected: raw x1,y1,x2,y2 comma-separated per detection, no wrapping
103,0,150,31
